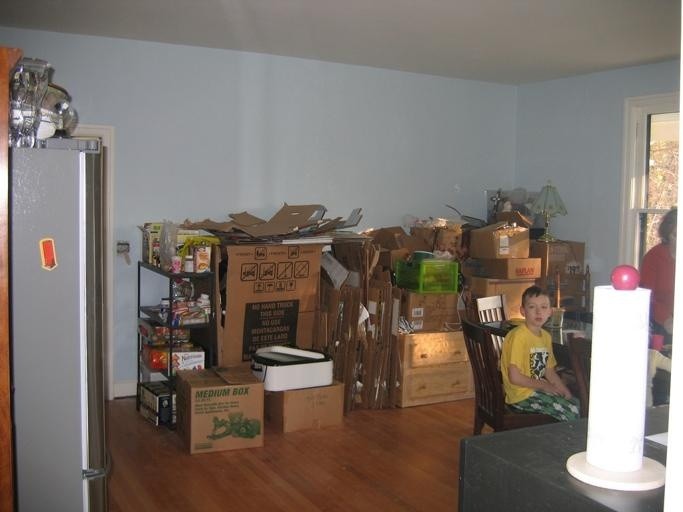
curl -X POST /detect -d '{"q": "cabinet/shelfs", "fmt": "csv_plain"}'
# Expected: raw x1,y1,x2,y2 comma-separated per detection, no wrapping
137,260,216,412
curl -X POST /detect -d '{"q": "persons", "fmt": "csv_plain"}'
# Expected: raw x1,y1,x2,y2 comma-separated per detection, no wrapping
500,286,581,422
640,207,677,407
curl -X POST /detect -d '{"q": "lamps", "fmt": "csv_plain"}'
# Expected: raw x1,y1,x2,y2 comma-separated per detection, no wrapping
566,283,665,492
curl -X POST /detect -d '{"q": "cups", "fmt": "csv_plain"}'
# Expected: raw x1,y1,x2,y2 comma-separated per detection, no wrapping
171,255,183,273
552,306,565,327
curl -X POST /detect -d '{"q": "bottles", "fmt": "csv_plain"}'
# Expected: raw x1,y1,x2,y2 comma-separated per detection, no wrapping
184,255,195,273
7,58,75,147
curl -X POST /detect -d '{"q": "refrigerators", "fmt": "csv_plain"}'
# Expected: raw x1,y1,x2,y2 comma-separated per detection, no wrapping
9,137,112,512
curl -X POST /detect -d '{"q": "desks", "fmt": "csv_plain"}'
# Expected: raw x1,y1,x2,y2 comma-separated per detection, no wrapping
459,402,669,511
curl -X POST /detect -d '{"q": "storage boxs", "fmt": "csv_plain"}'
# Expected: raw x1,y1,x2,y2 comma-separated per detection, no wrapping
139,381,177,427
136,222,163,264
171,347,205,374
142,344,169,368
